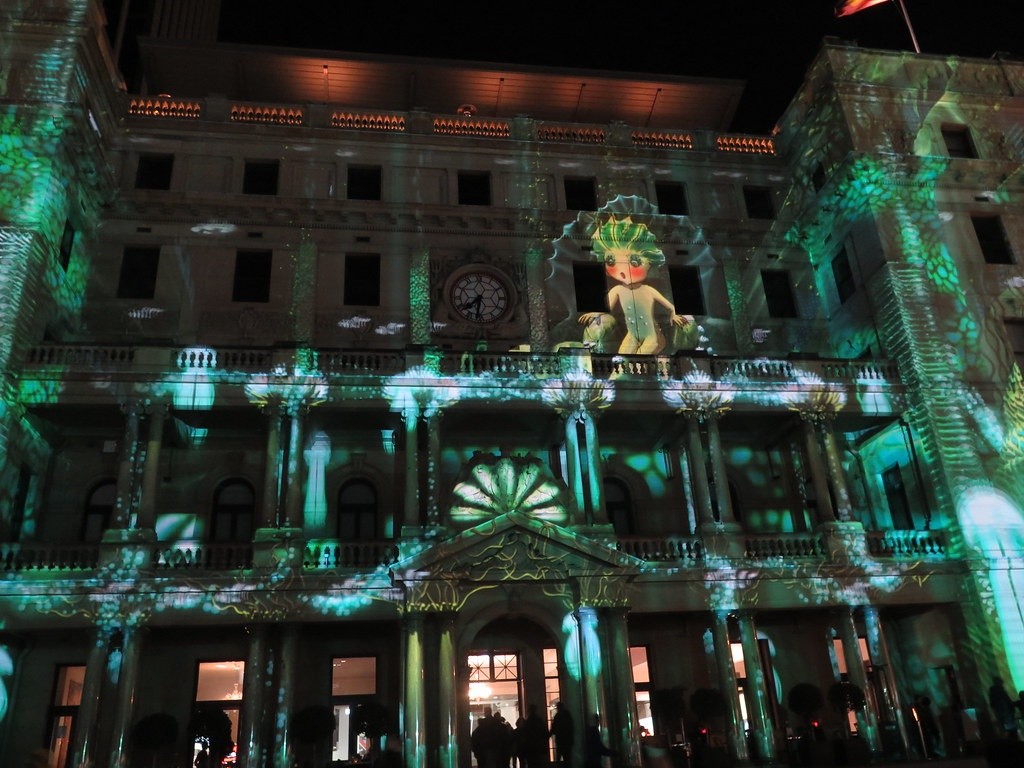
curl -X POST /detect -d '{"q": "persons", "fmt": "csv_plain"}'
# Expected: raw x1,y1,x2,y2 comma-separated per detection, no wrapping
470,703,615,768
905,675,1024,768
195,742,208,768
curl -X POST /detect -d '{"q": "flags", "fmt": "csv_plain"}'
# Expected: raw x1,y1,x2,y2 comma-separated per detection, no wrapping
834,0,890,18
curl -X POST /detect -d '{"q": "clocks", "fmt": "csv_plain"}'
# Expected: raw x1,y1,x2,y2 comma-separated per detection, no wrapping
451,273,509,323
